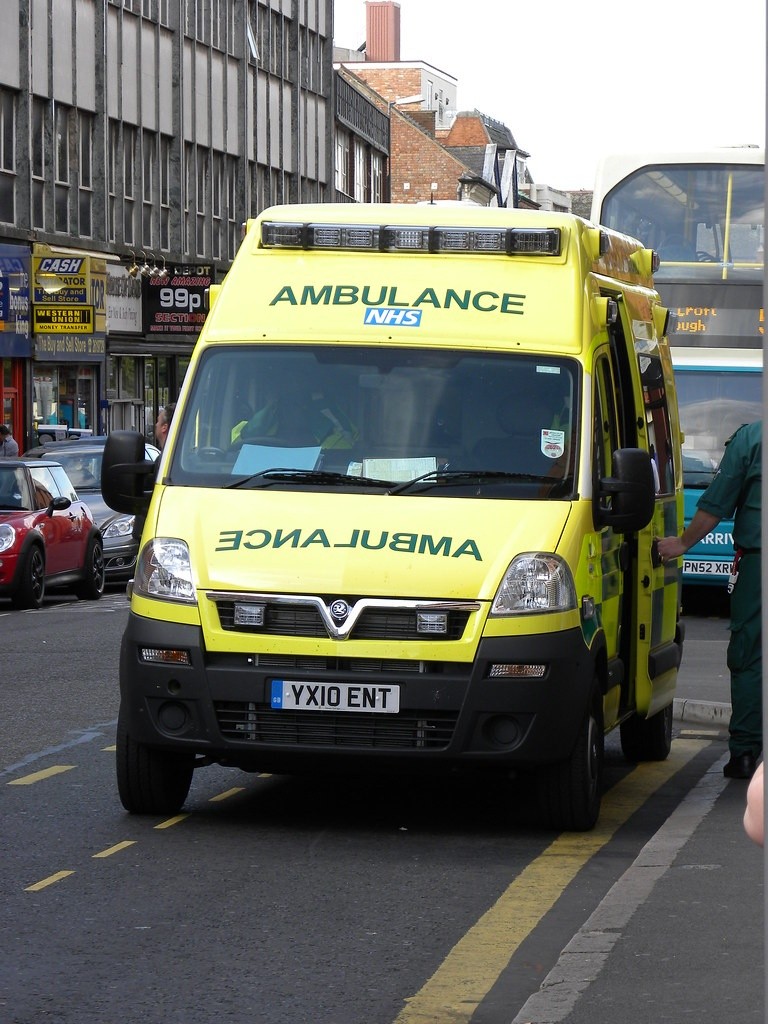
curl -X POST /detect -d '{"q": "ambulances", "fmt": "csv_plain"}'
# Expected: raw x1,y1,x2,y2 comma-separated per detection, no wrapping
98,182,684,814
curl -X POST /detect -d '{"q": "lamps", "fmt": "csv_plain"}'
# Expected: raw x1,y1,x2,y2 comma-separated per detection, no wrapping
140,253,159,279
120,249,139,277
150,254,167,279
130,250,150,277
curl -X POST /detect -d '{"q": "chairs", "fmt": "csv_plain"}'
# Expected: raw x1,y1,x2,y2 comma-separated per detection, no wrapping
655,234,698,262
472,394,555,483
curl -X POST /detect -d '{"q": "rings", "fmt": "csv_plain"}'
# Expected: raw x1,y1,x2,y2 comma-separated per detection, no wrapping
658,552,661,556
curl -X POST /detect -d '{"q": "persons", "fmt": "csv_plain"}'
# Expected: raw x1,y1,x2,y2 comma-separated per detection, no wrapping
0,425,18,457
665,440,674,493
230,375,360,450
657,410,764,775
133,403,183,543
744,760,764,848
650,443,660,493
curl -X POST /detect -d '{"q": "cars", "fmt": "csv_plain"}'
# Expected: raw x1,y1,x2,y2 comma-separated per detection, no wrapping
0,456,106,609
19,435,165,590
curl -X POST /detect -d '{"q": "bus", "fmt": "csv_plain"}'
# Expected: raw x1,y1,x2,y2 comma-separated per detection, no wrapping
586,141,768,614
